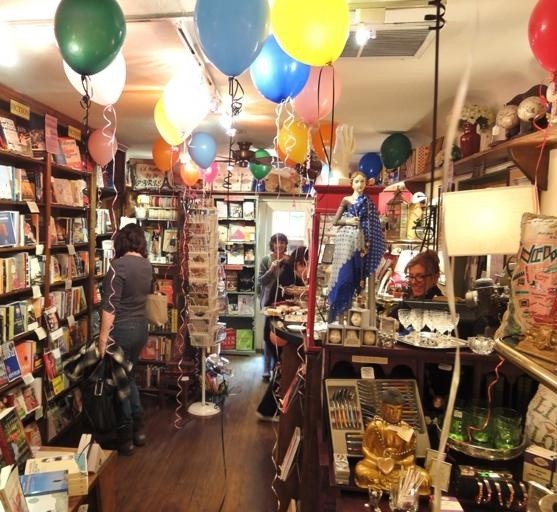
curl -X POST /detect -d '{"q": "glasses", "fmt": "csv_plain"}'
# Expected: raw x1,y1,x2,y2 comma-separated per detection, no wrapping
404,273,433,283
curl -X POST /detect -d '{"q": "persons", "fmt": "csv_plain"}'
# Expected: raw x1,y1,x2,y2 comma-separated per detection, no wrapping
332,170,384,315
254,246,311,422
394,248,445,319
257,234,293,380
354,386,430,493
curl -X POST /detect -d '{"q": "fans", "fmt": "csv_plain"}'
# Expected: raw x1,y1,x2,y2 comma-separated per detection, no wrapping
215,141,280,167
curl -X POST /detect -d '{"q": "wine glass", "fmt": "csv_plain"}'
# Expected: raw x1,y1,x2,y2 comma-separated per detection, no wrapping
396,307,459,346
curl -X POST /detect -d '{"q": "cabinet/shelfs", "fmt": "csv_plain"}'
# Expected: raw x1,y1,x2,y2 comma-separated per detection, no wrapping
268,126,557,512
0,83,259,475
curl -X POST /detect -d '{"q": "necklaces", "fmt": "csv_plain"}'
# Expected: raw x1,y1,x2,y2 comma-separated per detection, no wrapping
95,223,153,454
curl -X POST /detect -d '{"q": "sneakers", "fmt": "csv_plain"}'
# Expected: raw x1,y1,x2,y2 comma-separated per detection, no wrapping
256,412,279,423
113,441,136,457
133,432,146,446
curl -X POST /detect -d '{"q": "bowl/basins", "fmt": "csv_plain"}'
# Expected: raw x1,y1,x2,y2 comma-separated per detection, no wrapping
468,336,494,355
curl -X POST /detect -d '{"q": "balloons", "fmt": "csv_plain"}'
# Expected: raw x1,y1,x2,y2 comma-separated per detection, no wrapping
528,0,555,87
356,152,381,178
152,67,340,190
380,132,413,171
60,50,124,106
54,1,124,78
88,127,119,166
265,1,351,66
250,36,309,103
194,1,268,78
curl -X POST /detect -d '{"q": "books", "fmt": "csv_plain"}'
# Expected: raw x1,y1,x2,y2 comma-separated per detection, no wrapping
214,200,256,352
133,221,184,393
0,117,112,512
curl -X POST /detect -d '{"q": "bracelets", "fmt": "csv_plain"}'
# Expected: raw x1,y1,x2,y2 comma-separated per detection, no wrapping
98,338,107,344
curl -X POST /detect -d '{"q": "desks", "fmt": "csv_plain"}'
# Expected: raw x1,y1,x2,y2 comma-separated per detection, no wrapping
0,444,119,512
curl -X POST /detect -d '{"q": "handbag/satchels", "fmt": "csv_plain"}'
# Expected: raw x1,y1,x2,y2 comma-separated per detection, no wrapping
79,358,118,432
147,273,168,328
